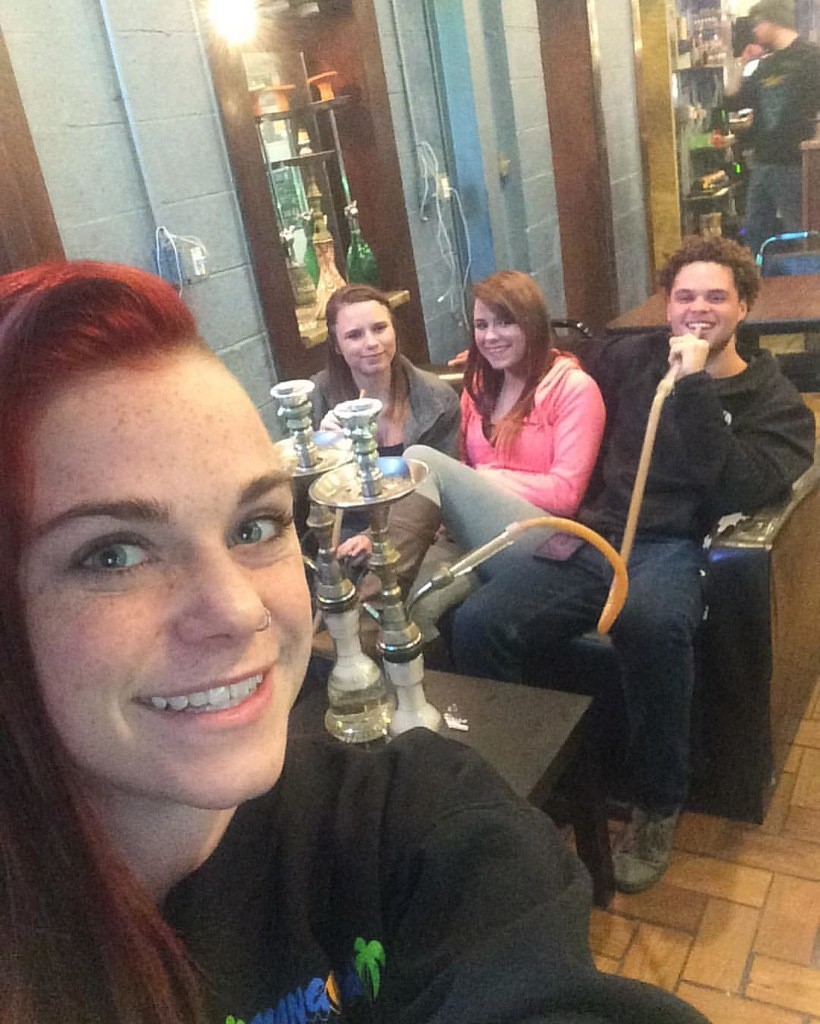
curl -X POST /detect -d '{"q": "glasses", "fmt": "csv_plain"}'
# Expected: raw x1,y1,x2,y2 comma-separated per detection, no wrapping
751,16,768,29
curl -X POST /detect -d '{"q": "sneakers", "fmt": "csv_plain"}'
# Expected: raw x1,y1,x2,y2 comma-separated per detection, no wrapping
611,793,693,891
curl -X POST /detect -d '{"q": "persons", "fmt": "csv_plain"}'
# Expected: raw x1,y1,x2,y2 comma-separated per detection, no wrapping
298,241,815,894
0,261,709,1024
720,0,820,259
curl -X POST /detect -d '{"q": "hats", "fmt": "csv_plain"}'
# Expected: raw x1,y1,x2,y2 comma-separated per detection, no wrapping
751,0,797,27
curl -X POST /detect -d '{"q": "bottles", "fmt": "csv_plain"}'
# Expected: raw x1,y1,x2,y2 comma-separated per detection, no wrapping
672,6,735,66
280,212,319,305
312,212,347,320
343,208,383,294
715,94,730,137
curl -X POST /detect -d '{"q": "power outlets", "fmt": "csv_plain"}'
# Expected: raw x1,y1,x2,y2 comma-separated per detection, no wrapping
167,239,209,288
425,169,452,206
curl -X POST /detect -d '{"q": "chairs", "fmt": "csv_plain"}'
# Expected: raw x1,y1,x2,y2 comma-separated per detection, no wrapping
753,230,820,279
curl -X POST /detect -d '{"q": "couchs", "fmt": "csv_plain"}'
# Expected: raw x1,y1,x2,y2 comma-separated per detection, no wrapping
438,370,819,827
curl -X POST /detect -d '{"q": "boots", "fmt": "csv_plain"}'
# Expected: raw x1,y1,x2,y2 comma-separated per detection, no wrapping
312,509,374,600
309,493,445,663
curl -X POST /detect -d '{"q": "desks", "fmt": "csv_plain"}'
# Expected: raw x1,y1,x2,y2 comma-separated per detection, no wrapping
604,273,819,335
289,653,618,909
